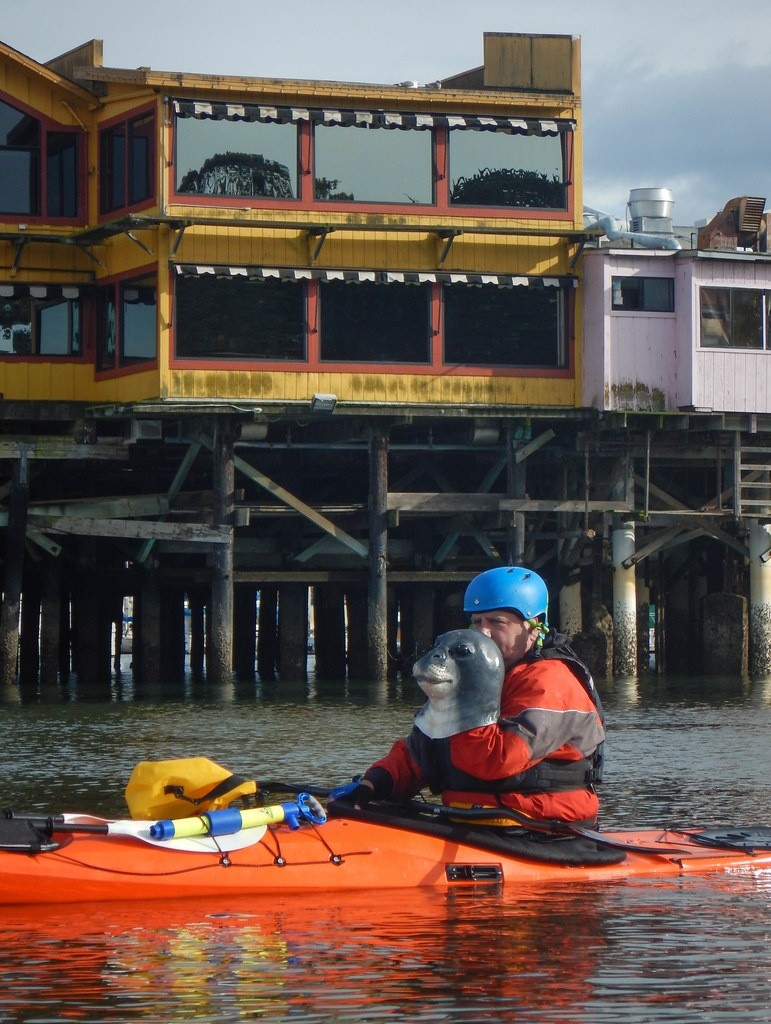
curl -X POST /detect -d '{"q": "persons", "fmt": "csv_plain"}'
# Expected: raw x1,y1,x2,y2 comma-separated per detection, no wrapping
325,566,605,836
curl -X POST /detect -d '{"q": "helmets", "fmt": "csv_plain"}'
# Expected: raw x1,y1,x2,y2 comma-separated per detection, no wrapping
463,566,549,640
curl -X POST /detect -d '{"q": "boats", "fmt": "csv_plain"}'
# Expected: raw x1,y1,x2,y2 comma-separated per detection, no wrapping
1,798,770,906
